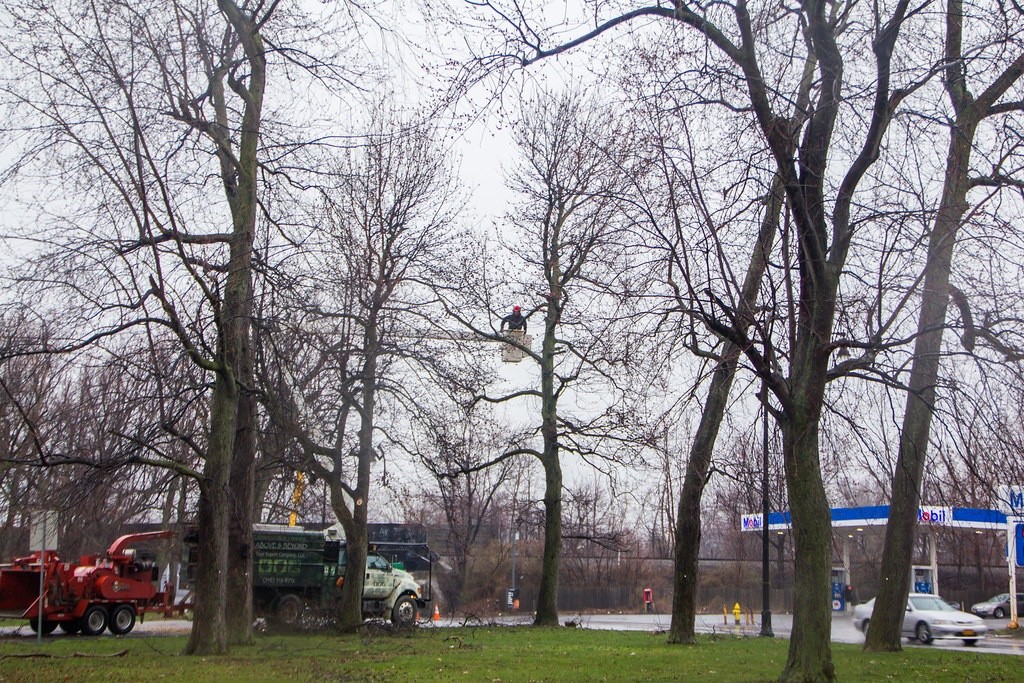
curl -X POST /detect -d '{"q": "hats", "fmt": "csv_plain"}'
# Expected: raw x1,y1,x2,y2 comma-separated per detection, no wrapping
513,305,521,311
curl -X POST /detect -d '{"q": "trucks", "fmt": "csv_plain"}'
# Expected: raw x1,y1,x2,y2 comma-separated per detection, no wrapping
246,522,425,630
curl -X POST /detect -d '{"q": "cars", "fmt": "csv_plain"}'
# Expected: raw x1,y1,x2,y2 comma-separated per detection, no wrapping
970,593,1024,619
852,592,987,646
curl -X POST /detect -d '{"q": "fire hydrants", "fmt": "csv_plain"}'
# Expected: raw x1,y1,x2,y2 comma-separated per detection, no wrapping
733,603,742,626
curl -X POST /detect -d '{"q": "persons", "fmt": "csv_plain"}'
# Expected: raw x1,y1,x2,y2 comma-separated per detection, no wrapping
500,305,528,335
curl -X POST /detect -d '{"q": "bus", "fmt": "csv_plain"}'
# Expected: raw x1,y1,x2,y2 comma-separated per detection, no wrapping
0,528,185,639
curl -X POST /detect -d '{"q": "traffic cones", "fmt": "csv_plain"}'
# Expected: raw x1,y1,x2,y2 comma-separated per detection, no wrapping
434,604,441,620
